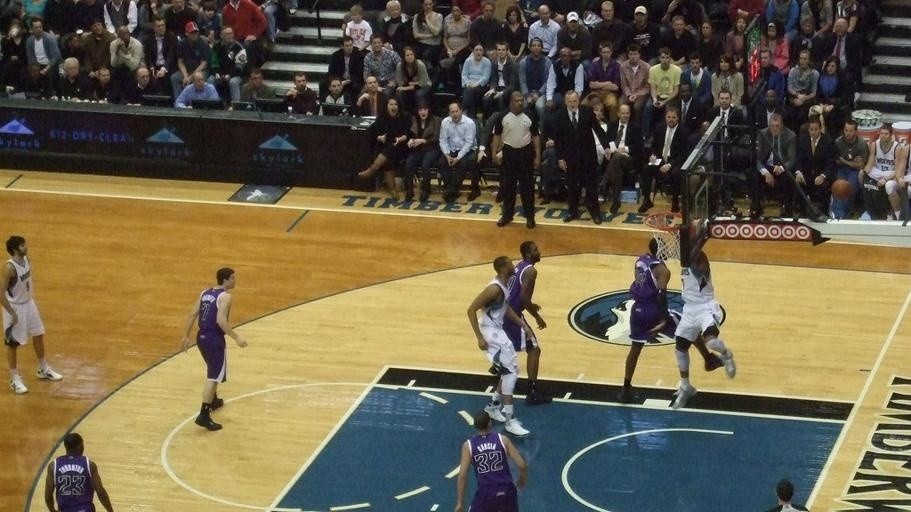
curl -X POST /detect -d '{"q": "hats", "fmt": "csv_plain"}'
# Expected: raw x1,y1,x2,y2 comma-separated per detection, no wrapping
186,22,200,33
566,12,579,22
634,6,646,15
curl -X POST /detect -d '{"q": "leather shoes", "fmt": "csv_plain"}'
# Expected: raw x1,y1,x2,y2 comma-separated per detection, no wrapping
442,188,681,228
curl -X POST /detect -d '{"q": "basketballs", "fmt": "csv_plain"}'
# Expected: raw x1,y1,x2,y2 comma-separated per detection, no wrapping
832,179,852,198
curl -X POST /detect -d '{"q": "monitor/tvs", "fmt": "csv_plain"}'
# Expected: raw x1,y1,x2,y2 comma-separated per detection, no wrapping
257,98,283,112
191,98,223,109
230,100,253,111
322,103,353,115
142,95,170,106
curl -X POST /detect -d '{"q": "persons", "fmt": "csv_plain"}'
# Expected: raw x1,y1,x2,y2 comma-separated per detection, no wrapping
467,256,532,437
622,238,723,401
673,230,736,408
455,410,527,511
44,434,114,511
0,235,62,394
769,479,808,511
1,0,911,229
179,268,247,430
489,241,551,406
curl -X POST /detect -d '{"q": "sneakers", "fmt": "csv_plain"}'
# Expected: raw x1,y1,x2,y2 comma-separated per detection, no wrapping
38,367,63,381
673,385,698,409
724,353,736,377
484,405,507,422
504,419,531,436
525,392,552,406
9,378,28,393
195,414,222,430
210,398,224,411
707,354,723,372
618,391,644,403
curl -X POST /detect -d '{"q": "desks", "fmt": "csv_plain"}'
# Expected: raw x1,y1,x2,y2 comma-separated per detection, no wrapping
0,96,209,182
201,108,379,193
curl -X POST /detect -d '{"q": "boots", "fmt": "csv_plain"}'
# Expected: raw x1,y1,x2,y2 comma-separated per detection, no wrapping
406,172,415,202
359,154,388,180
420,170,432,202
384,170,401,201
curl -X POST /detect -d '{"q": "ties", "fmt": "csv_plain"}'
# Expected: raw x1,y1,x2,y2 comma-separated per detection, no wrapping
663,127,673,164
774,133,779,164
614,125,624,148
835,36,842,57
571,111,577,127
